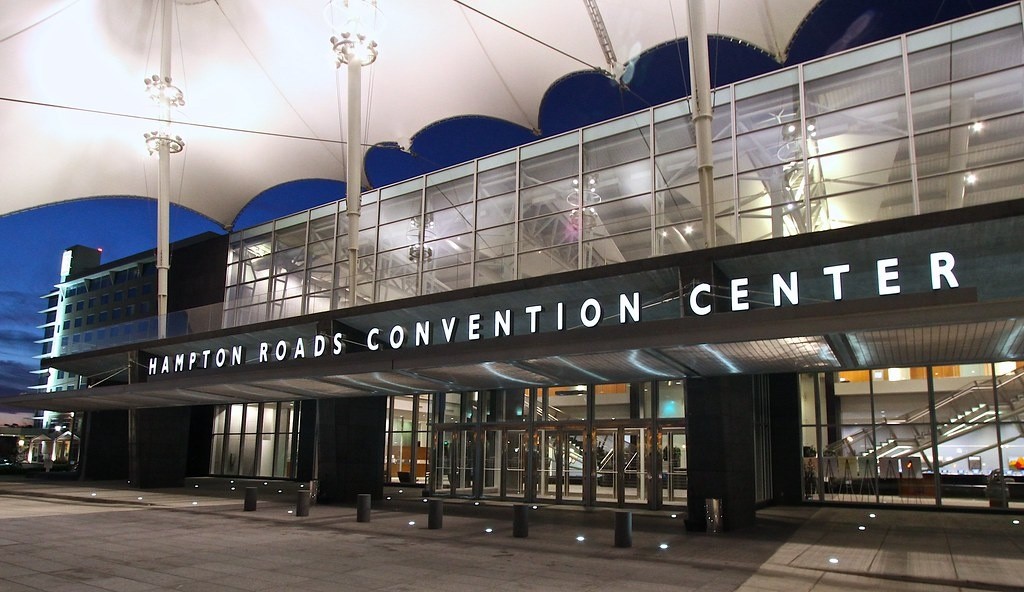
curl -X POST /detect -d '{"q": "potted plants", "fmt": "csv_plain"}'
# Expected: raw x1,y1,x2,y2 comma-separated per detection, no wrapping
986,469,1010,508
803,461,817,493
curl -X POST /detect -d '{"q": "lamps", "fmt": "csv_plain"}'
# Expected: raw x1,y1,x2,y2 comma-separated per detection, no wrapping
784,117,816,209
408,214,436,263
330,31,378,67
140,73,186,155
570,175,600,228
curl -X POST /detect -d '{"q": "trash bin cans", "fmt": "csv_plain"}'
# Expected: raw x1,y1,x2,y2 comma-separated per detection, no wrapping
705,498,723,534
310,480,319,505
986,486,1009,507
